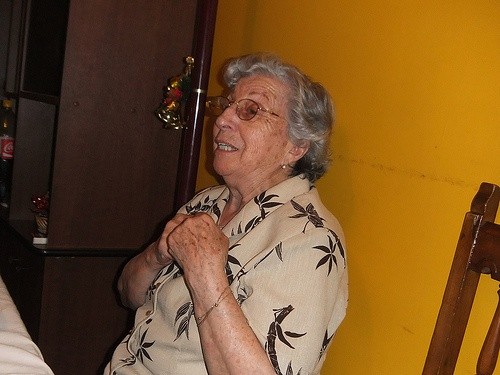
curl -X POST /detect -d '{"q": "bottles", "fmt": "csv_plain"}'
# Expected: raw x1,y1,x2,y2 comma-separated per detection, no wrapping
0,100,16,201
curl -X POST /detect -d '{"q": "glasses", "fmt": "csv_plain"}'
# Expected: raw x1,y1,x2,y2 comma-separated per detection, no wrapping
206,96,286,121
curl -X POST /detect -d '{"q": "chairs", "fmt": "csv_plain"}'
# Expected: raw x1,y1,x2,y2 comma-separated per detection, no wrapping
422,182,500,375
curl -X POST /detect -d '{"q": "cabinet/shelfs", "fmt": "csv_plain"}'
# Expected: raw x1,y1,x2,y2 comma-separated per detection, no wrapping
0,0,218,375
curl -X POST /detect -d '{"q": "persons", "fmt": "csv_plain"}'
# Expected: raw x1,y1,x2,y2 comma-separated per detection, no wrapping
100,54,349,375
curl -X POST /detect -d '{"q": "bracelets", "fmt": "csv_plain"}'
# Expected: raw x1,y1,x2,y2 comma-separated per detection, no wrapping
196,287,232,327
